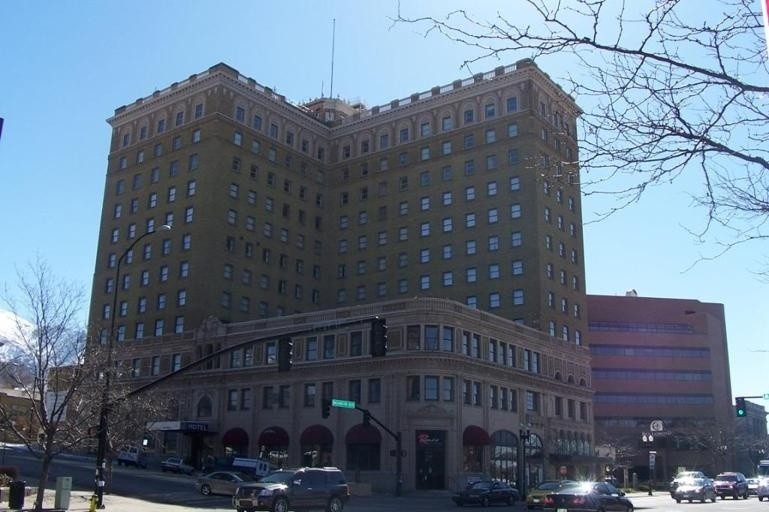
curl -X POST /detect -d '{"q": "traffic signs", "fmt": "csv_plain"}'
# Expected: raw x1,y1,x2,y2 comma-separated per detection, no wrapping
333,399,356,409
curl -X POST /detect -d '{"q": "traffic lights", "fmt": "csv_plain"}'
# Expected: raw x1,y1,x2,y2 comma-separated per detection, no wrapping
363,409,371,425
370,319,388,357
278,336,293,371
736,397,746,417
322,399,330,418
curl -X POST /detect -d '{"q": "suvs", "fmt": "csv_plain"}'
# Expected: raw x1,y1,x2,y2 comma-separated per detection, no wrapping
231,467,352,512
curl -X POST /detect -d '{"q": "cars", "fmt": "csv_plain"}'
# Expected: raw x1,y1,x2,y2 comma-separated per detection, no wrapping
528,480,634,512
452,480,520,507
195,471,256,496
670,470,757,503
161,457,194,474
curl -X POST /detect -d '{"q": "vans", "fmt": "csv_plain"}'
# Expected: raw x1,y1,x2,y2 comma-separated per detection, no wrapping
117,446,147,468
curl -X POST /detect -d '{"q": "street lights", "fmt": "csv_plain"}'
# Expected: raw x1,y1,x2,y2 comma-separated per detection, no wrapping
519,422,532,501
96,225,172,505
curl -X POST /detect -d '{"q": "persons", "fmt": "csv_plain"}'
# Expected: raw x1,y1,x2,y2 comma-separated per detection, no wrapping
201,456,215,474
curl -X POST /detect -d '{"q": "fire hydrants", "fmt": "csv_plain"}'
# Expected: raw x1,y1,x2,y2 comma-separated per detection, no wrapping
89,495,99,511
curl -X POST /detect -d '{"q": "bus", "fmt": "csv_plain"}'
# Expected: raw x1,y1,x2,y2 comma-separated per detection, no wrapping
758,460,769,500
